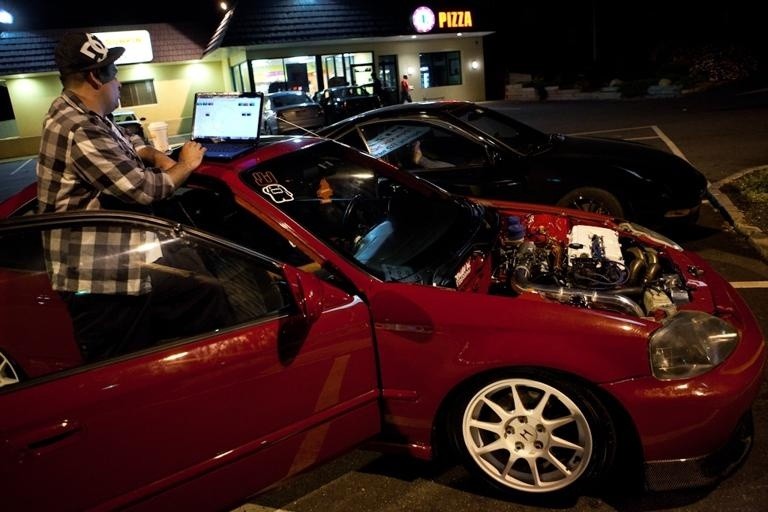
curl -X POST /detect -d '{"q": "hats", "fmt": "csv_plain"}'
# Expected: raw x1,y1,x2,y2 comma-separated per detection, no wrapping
54,31,125,72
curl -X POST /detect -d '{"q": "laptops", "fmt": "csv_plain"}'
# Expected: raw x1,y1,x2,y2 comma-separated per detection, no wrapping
169,91,264,162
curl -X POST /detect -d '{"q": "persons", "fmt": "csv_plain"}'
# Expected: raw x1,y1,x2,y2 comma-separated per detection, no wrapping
34,26,237,363
398,75,413,104
404,140,457,168
